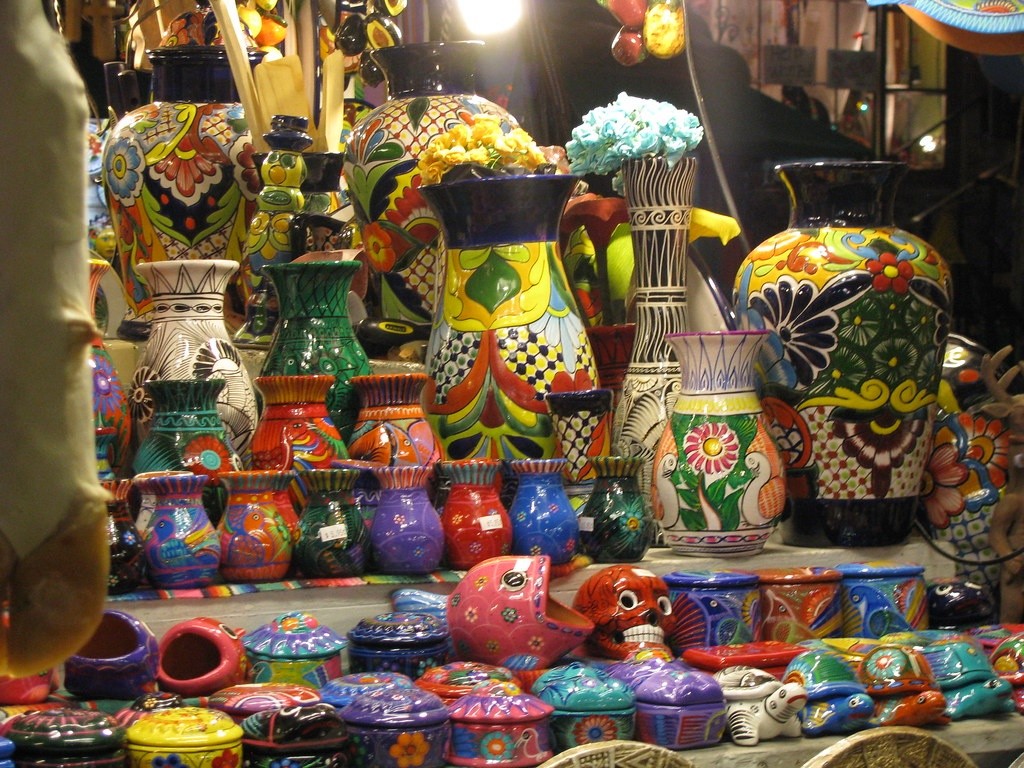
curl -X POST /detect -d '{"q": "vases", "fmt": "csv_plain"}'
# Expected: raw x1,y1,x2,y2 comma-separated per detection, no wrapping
89,43,1005,594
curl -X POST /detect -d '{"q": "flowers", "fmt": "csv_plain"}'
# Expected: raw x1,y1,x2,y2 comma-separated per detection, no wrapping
589,205,741,324
562,196,630,325
418,114,546,187
565,91,704,196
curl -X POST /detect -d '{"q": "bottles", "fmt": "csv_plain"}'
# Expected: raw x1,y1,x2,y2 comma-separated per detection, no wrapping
95,428,117,479
128,378,245,478
130,471,221,589
579,455,655,563
241,374,351,471
329,460,445,576
499,458,578,567
347,372,448,467
215,467,299,583
293,468,372,578
97,478,146,596
434,461,513,571
